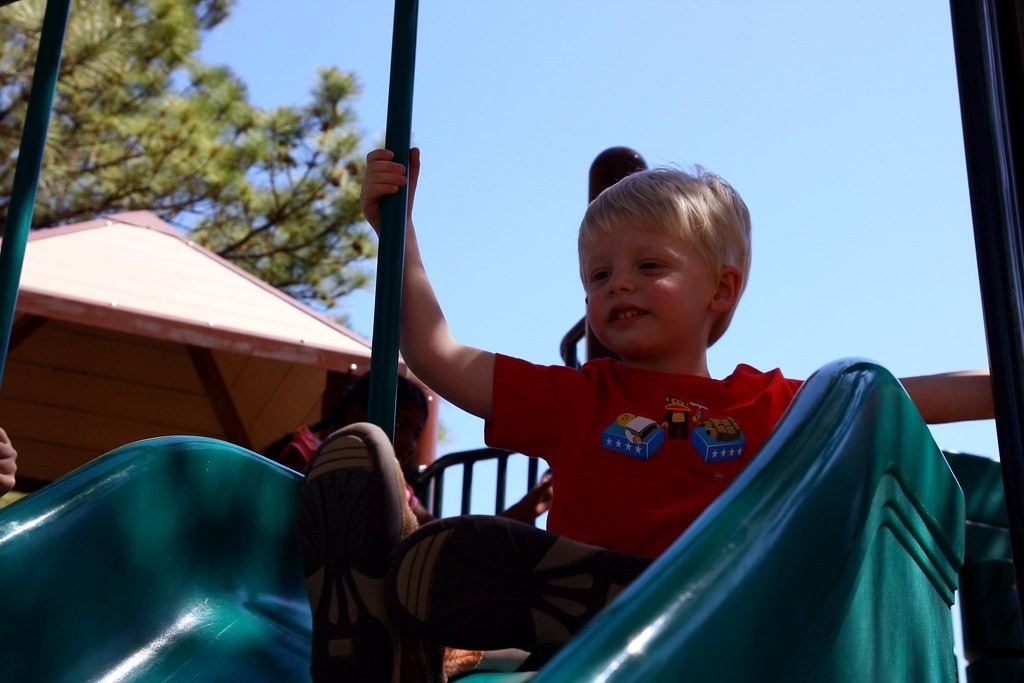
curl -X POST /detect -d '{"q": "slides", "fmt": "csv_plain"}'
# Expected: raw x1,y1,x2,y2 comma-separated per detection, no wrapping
0,354,966,683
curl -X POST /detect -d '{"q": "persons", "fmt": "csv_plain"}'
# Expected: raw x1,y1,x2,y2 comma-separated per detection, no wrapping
0,428,18,499
263,147,994,683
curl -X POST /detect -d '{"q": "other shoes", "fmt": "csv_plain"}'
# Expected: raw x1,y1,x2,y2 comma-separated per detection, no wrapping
293,421,453,683
383,514,659,655
